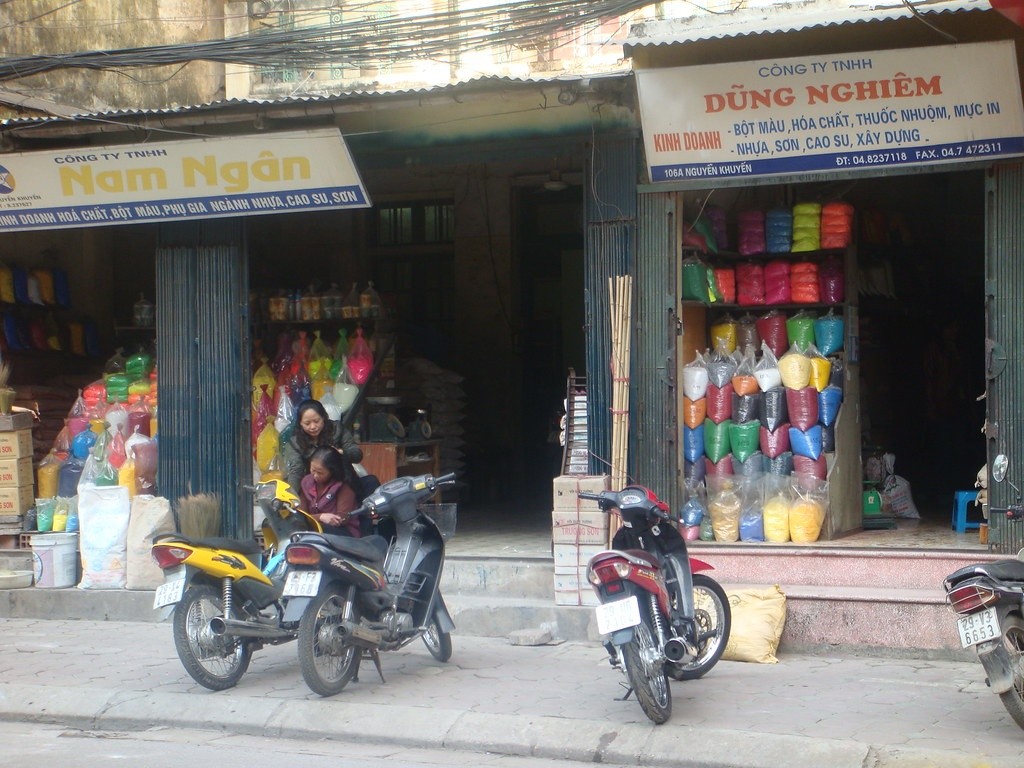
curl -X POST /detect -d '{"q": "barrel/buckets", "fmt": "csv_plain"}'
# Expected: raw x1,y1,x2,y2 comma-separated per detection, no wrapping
29,533,77,589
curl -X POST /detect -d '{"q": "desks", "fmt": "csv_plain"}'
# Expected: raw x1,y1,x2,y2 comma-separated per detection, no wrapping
357,438,446,531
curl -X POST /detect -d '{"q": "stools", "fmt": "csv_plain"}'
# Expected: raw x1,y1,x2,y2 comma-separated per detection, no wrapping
950,490,988,534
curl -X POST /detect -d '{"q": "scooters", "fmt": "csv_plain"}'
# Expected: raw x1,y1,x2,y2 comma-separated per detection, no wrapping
151,479,334,692
944,455,1024,730
278,469,462,700
573,485,733,726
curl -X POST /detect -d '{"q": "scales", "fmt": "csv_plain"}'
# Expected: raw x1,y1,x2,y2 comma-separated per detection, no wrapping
862,481,882,519
407,407,433,443
364,395,407,444
862,514,897,530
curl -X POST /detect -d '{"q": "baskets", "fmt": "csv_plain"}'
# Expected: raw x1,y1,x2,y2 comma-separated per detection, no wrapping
417,503,457,536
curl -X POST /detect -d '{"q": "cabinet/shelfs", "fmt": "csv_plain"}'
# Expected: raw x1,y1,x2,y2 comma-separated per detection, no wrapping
0,304,111,360
688,243,864,544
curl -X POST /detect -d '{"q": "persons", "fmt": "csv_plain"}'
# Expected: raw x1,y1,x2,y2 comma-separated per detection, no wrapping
280,399,396,547
922,304,978,493
298,444,361,539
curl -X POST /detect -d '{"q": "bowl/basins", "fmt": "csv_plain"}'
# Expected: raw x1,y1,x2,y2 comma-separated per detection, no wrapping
0,570,34,590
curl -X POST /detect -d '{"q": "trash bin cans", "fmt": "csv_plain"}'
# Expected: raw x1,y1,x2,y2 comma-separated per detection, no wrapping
29,532,78,589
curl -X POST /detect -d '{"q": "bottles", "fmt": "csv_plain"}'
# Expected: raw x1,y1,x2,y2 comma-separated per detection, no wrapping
352,418,361,444
266,279,379,321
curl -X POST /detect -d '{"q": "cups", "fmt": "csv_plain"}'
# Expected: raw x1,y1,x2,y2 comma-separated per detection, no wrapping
0,388,16,415
132,293,155,326
361,307,370,317
353,307,360,318
371,304,379,317
341,306,353,318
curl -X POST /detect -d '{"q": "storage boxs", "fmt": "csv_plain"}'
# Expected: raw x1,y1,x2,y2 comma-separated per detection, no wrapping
0,411,35,516
552,475,611,512
553,574,600,607
552,512,610,545
553,543,609,573
